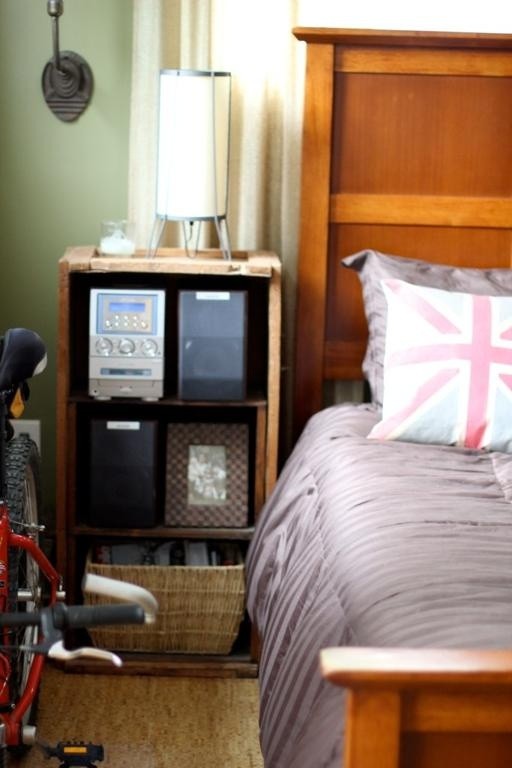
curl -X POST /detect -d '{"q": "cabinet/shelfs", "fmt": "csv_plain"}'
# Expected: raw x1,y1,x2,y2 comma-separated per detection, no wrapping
47,243,285,685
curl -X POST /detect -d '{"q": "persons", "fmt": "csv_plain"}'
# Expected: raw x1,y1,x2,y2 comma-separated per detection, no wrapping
190,451,224,498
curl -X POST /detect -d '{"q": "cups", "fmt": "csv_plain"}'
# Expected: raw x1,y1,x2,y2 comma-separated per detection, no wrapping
101,220,135,256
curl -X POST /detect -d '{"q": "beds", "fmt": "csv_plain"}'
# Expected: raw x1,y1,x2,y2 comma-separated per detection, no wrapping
238,21,512,766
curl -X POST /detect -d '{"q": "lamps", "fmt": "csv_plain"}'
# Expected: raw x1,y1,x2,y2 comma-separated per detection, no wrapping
144,65,236,260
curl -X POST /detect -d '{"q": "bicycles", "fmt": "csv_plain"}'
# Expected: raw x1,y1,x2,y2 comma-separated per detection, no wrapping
0,319,155,766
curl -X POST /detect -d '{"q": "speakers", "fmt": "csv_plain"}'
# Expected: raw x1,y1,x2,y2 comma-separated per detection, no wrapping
89,420,157,528
178,289,246,400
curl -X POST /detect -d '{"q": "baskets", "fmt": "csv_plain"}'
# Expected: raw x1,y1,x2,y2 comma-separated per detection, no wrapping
77,542,245,657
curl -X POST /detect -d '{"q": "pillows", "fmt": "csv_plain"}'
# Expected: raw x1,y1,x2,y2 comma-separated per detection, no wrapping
338,245,511,417
362,274,511,456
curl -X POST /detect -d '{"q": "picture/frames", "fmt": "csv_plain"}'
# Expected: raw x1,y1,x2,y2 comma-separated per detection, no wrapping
161,420,252,529
